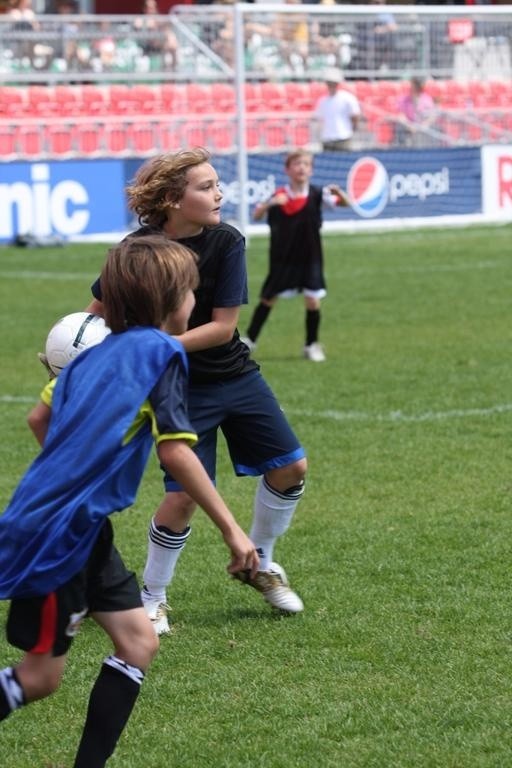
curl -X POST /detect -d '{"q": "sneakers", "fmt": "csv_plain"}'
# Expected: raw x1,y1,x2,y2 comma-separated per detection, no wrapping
140,588,171,635
227,562,304,612
239,335,256,353
304,342,325,362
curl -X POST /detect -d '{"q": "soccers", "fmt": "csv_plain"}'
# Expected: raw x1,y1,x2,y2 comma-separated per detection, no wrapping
46,312,112,377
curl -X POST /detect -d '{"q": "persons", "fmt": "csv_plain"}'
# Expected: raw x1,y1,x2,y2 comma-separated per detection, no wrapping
394,76,438,146
311,67,361,150
